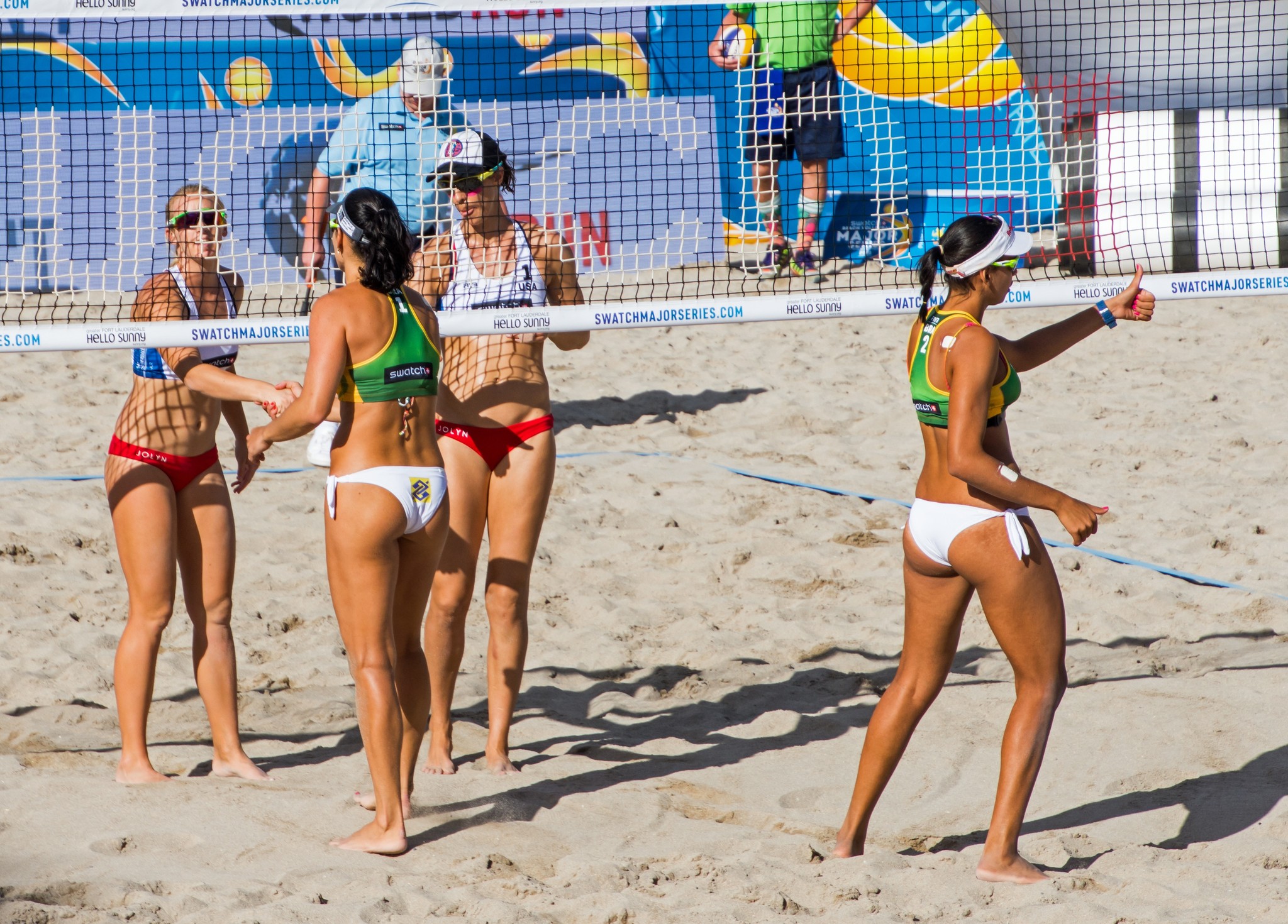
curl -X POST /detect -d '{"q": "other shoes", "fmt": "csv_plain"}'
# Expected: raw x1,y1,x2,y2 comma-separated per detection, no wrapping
307,418,343,468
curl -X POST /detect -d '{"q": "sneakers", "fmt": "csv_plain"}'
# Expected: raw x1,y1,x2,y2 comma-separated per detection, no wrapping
758,239,793,277
790,249,825,283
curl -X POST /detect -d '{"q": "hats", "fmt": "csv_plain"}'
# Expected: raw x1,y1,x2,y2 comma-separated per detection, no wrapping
399,36,444,95
426,129,502,183
941,215,1033,279
325,187,372,245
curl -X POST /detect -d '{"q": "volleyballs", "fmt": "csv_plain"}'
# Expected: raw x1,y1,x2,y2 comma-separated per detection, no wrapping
718,23,761,69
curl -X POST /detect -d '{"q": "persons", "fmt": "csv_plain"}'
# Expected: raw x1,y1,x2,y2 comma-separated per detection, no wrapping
403,129,591,776
833,216,1155,885
302,36,509,468
105,184,295,785
244,188,443,855
708,0,877,283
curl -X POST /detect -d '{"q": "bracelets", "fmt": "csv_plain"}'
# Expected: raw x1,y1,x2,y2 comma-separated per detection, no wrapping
1096,300,1118,329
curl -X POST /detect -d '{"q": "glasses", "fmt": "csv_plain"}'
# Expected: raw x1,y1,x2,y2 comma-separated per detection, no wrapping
329,217,341,232
166,208,228,231
438,159,503,197
990,254,1021,274
